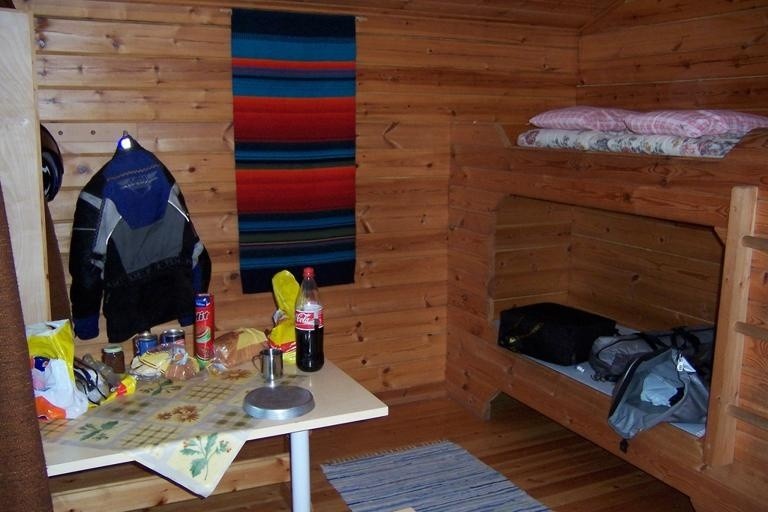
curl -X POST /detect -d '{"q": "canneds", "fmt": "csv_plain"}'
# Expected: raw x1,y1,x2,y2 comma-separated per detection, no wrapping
134,333,158,355
162,328,185,350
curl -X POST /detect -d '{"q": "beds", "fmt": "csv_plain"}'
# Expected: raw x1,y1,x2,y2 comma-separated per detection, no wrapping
445,117,768,512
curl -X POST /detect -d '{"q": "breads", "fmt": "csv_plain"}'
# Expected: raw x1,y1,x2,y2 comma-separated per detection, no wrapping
212,329,270,368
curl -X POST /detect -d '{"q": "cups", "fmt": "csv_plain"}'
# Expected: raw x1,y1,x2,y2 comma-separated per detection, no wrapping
251,349,283,382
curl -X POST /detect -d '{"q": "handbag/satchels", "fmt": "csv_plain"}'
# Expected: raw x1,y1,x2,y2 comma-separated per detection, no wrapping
25,318,88,424
588,328,715,454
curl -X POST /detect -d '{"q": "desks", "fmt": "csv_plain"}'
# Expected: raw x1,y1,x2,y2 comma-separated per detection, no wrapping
39,353,389,511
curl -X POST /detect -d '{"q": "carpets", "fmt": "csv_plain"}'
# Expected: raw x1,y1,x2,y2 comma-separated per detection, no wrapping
317,437,555,512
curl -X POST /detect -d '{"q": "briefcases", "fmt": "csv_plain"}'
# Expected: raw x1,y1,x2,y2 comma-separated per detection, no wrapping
497,301,620,366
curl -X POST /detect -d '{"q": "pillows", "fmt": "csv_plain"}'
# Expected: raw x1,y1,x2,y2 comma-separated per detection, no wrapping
528,106,768,140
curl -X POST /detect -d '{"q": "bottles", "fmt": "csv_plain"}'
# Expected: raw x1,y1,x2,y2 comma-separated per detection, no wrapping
295,267,325,371
